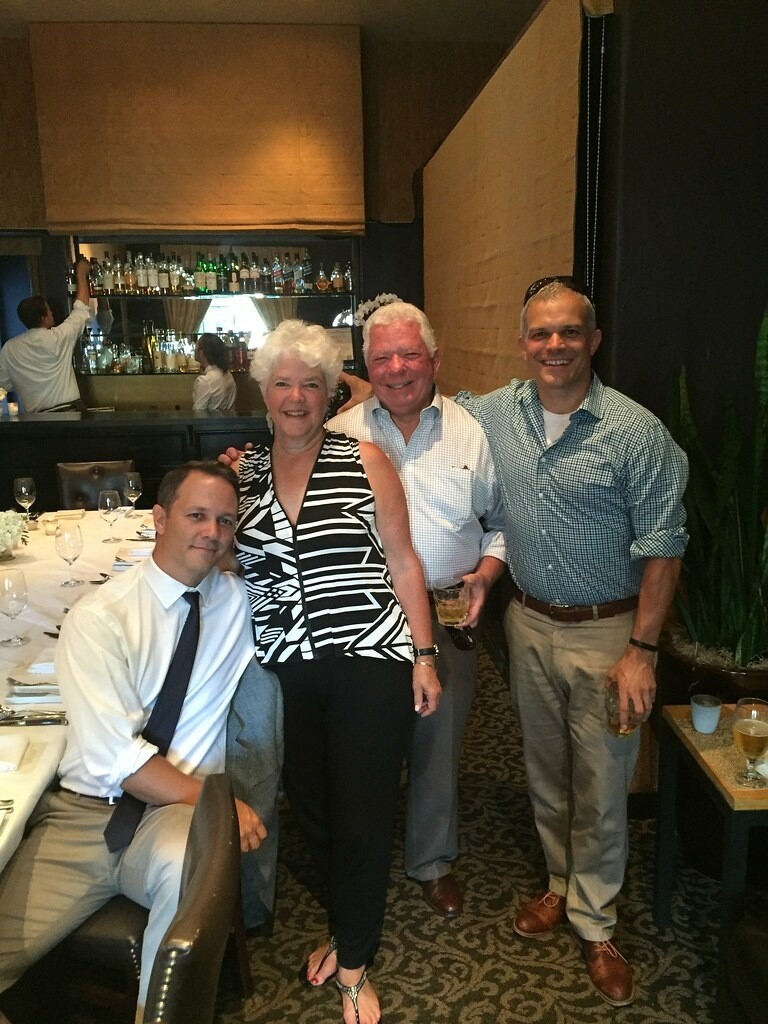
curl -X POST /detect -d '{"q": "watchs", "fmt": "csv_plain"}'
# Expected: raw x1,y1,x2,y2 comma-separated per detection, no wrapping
414,644,439,660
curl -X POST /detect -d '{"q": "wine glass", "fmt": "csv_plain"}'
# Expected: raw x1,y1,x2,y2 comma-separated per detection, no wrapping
0,568,31,647
14,478,37,523
98,490,122,543
55,525,84,587
122,472,143,519
732,698,768,789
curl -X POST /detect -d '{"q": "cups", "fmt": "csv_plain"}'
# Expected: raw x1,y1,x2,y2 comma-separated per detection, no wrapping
7,403,19,415
690,694,722,734
42,519,58,535
432,577,468,627
604,683,637,739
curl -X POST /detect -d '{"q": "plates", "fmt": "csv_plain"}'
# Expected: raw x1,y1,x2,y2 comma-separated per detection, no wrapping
5,684,61,704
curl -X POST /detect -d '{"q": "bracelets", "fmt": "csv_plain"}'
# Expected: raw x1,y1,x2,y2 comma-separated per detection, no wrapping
414,662,437,672
629,636,658,652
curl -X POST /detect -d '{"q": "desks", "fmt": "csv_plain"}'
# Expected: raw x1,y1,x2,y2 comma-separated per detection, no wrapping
0,510,159,871
653,704,768,1024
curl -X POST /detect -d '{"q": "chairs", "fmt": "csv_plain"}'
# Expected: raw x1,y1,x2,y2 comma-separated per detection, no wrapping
55,459,134,511
57,654,285,1024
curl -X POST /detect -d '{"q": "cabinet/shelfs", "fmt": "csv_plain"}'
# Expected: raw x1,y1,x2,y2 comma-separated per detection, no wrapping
63,234,362,376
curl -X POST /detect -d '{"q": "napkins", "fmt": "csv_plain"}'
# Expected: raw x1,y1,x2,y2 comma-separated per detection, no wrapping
0,735,29,771
4,662,62,703
55,509,85,520
112,561,138,571
128,549,153,556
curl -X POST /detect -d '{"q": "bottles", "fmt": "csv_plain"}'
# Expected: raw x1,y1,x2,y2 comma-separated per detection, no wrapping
78,319,250,375
65,251,352,295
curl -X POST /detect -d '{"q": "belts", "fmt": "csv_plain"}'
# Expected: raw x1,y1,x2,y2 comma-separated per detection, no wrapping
514,588,642,624
37,398,85,412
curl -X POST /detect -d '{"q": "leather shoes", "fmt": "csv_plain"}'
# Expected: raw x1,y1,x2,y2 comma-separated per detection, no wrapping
412,879,464,919
512,889,565,938
571,931,637,1006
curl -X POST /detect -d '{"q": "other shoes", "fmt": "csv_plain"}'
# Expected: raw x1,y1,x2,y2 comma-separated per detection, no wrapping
302,937,341,986
335,965,382,1024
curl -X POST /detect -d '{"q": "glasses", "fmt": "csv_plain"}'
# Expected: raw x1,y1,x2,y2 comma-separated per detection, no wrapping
523,275,594,307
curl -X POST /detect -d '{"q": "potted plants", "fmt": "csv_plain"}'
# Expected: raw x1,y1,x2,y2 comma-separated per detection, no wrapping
658,307,767,711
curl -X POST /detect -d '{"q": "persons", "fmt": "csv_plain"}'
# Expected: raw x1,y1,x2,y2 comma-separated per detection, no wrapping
0,260,91,416
337,283,690,1006
190,333,237,411
217,301,508,916
1,460,268,1023
218,317,441,1024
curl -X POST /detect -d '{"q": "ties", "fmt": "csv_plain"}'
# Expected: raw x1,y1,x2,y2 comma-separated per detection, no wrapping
102,589,201,853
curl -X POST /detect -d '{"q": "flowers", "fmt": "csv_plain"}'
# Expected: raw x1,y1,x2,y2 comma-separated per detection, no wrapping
0,510,30,556
